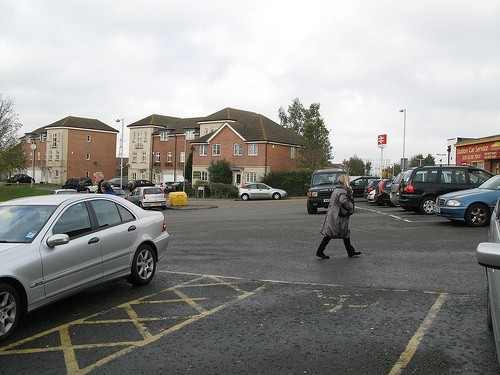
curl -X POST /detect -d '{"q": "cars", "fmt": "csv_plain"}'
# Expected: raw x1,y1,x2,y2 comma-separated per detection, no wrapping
363,179,400,207
108,178,129,189
475,191,500,370
390,164,494,215
51,185,132,198
7,174,35,186
129,180,155,192
0,195,172,344
349,176,385,196
124,187,167,209
435,175,500,225
305,167,354,214
237,182,287,200
166,182,193,193
61,176,92,193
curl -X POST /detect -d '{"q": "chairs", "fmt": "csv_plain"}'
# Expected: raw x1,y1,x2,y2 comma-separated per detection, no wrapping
18,207,45,231
457,173,465,183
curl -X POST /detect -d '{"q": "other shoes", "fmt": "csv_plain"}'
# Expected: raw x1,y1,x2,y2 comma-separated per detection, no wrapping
348,252,361,257
316,252,329,259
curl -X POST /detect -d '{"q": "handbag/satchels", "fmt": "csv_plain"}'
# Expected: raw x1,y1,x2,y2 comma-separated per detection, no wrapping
338,193,354,217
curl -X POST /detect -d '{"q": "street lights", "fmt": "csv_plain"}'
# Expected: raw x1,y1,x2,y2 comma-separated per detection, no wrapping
31,142,37,185
168,138,187,189
116,117,124,189
399,108,407,170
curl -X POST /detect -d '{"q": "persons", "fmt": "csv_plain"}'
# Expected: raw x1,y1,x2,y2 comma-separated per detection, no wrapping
93,171,113,194
316,174,361,259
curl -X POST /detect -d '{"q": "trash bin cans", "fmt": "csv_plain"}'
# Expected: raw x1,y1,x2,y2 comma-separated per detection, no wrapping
169,192,187,206
